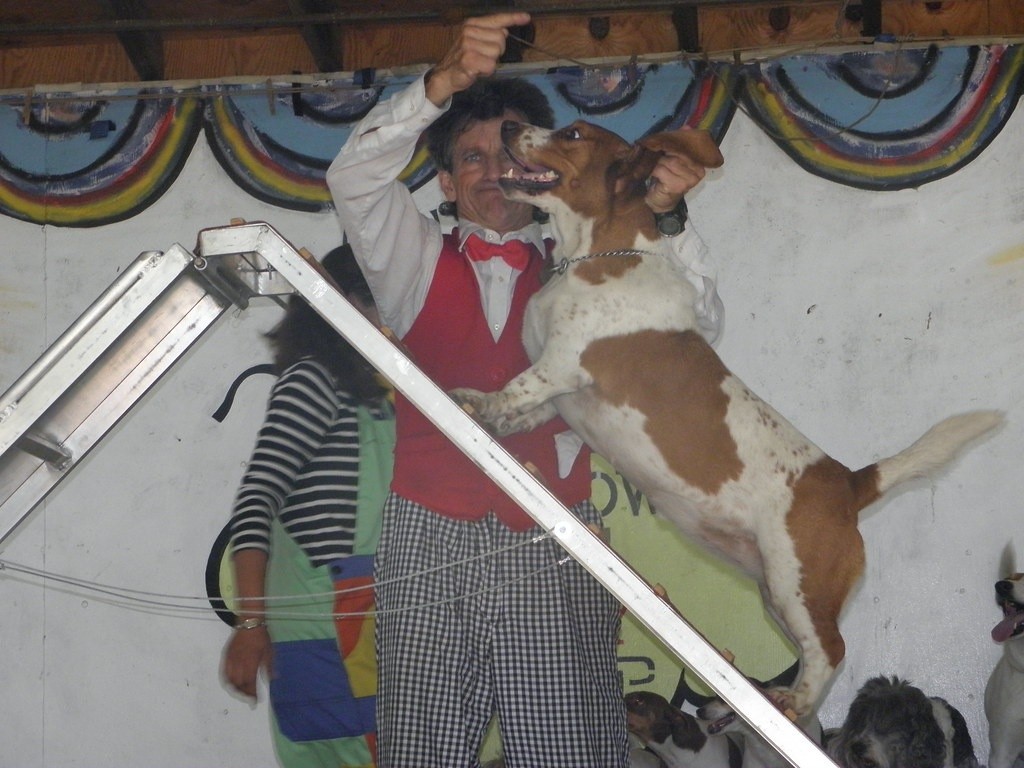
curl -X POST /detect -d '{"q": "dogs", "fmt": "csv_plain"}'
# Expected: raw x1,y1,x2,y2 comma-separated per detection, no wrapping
448,117,1006,722
977,546,1024,767
616,681,980,767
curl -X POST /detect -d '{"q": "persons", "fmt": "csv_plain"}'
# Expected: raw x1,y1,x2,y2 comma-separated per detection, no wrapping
218,243,398,768
322,7,707,767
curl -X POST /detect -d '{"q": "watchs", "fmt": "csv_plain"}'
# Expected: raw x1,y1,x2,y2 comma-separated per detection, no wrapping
653,201,691,240
233,616,266,632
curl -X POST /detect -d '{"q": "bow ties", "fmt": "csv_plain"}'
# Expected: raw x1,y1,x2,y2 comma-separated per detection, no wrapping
464,233,530,271
